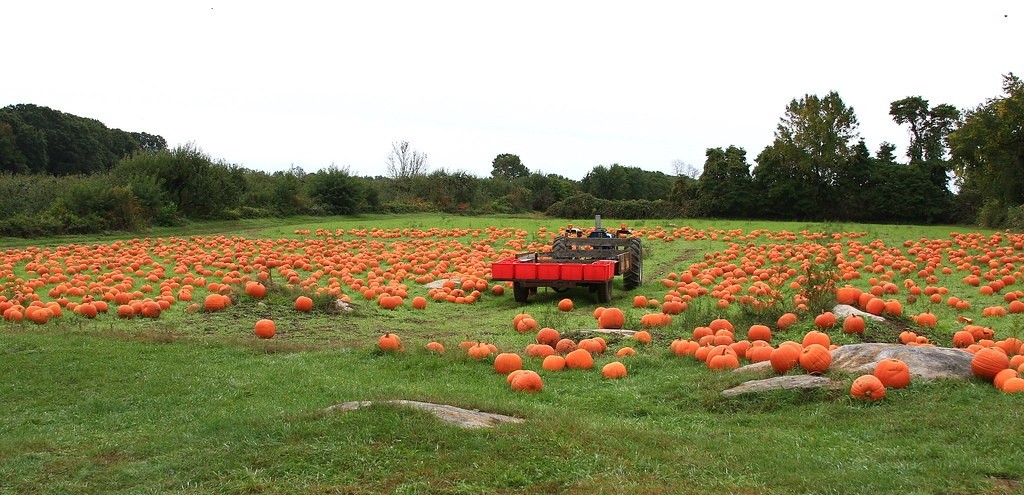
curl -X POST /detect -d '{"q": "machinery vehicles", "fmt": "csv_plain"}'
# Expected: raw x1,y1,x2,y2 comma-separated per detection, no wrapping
491,214,643,303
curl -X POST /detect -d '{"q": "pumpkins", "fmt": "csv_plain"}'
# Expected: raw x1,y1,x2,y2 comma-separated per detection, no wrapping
0,225,1024,401
